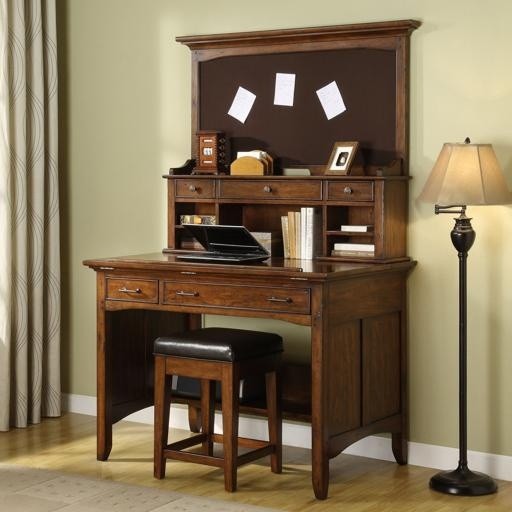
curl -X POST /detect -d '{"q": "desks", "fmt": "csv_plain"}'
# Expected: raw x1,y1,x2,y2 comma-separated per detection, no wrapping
82,252,418,501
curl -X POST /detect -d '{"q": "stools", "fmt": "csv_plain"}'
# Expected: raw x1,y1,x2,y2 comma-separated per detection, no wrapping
151,327,283,492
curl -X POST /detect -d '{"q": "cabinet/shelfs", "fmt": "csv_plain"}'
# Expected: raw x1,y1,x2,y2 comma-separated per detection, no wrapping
168,179,409,264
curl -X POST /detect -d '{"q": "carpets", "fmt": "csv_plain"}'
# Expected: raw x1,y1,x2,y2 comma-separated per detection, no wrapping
0,464,280,512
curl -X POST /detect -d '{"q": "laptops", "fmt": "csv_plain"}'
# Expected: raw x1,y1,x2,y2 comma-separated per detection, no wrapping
175,223,271,264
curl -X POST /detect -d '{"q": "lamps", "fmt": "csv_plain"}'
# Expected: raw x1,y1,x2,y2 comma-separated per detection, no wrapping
414,137,511,497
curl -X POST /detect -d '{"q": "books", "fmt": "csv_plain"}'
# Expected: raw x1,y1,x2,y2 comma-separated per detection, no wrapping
331,250,375,257
279,206,322,261
333,241,375,252
340,224,374,232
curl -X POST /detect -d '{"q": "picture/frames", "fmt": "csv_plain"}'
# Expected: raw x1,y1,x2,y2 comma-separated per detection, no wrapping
324,141,361,176
